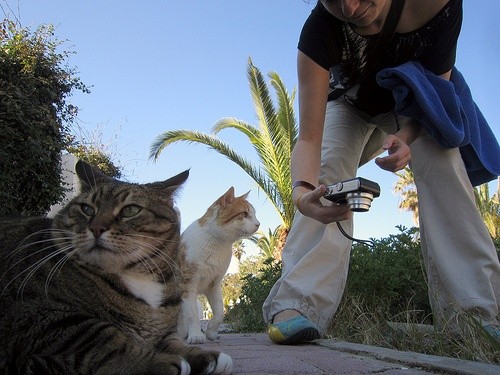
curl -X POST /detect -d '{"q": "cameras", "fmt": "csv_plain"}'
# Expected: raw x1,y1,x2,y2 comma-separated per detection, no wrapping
323,177,380,212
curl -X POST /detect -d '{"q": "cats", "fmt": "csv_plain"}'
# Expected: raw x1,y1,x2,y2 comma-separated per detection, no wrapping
180,186,260,344
0,158,233,375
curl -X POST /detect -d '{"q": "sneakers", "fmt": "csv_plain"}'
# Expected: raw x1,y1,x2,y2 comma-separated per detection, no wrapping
267,313,321,343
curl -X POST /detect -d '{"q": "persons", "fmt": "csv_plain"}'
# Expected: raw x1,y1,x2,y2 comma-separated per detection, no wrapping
262,0,500,345
